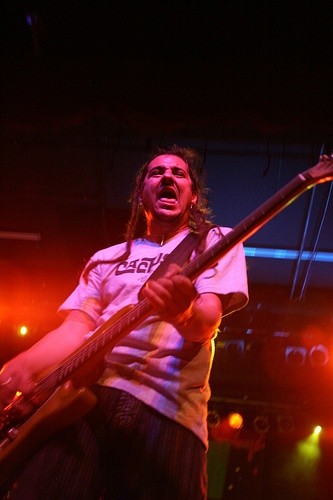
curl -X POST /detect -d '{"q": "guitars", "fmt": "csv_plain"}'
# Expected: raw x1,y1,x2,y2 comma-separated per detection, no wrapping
1,154,333,500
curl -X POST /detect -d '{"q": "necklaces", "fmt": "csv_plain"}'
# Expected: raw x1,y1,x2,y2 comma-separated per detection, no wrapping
146,225,190,249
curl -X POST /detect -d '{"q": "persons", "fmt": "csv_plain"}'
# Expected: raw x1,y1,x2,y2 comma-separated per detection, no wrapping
0,147,249,500
